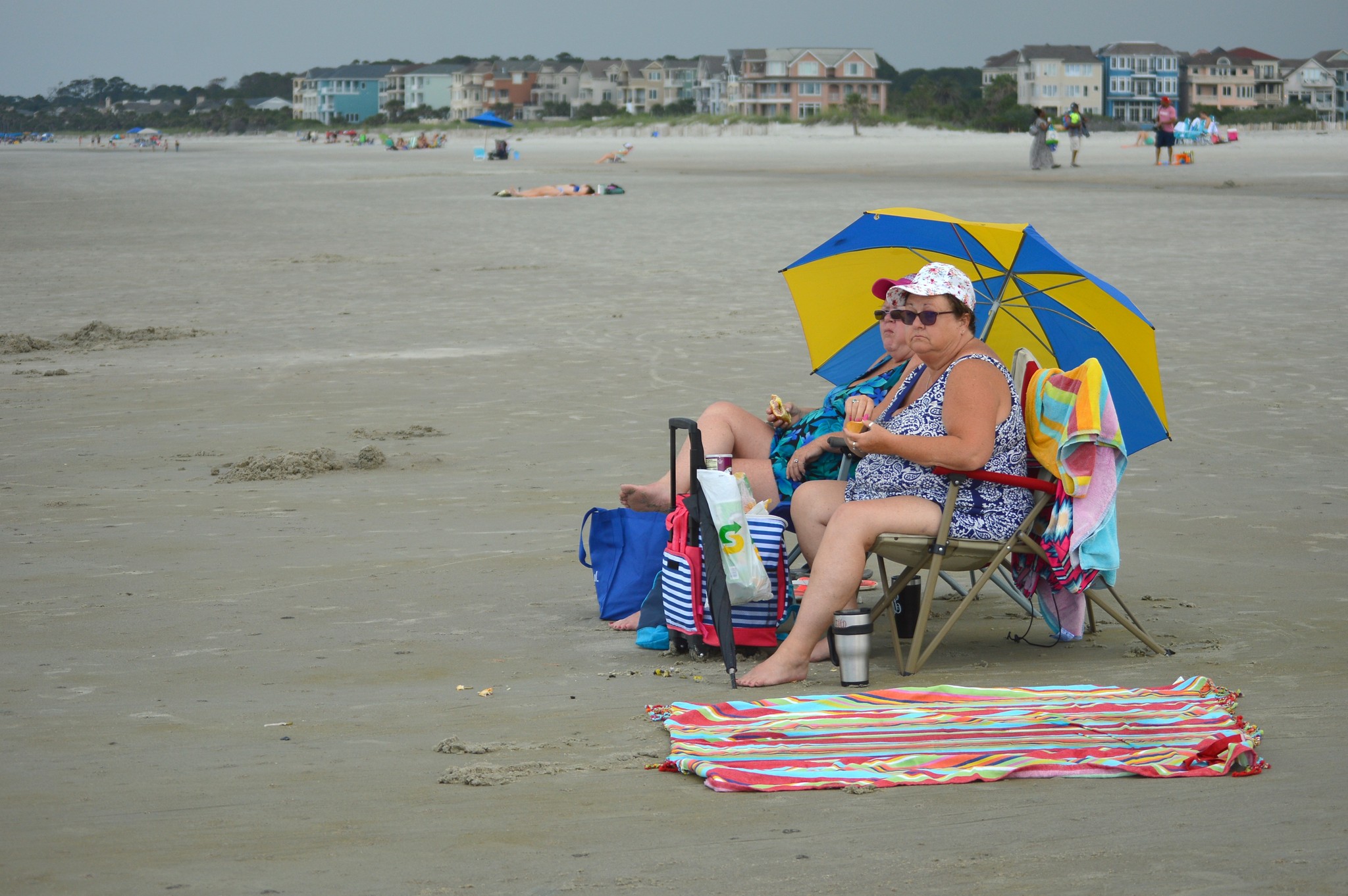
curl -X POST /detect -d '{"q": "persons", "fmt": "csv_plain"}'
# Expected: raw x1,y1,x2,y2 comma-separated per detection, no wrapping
301,131,366,145
596,143,633,164
1135,131,1155,146
487,141,507,160
506,183,594,197
1029,107,1061,170
78,134,179,153
386,132,446,150
1193,111,1212,144
1067,103,1089,167
609,262,1034,685
1154,96,1176,164
4,136,22,145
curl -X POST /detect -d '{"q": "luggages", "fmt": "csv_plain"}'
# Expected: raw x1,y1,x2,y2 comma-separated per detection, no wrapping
661,415,787,661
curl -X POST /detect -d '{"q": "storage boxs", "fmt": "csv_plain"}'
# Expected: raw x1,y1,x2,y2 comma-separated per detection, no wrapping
1227,129,1238,142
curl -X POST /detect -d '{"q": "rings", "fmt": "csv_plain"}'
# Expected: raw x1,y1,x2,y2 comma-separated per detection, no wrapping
852,399,859,404
852,442,855,450
869,422,874,428
793,459,798,463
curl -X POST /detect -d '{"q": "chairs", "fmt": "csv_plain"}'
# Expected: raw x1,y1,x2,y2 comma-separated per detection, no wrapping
787,346,1165,679
1174,114,1216,144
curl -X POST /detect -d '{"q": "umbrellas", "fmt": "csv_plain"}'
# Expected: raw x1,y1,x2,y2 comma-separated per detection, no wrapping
127,127,161,135
778,208,1174,455
0,132,53,138
113,134,125,140
682,428,739,688
465,111,513,152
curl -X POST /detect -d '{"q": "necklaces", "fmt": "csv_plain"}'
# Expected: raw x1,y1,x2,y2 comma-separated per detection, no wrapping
926,375,930,391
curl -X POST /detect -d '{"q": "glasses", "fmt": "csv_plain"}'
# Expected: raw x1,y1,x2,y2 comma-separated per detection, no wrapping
899,310,962,326
873,310,905,320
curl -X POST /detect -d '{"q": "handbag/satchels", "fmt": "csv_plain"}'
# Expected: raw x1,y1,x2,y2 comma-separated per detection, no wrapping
577,509,672,619
695,464,774,605
1046,122,1058,145
1030,125,1038,135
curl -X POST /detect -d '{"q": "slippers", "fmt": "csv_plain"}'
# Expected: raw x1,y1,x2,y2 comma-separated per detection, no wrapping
790,563,811,579
794,584,809,600
795,568,873,581
797,576,878,591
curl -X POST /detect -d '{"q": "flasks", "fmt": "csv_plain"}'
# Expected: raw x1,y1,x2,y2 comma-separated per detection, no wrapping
826,607,874,687
891,574,921,638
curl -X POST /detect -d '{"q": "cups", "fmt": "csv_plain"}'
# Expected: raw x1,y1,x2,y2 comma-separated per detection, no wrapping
705,454,733,475
712,498,751,584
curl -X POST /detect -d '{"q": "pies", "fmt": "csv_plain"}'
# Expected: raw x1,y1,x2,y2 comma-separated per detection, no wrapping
769,394,791,425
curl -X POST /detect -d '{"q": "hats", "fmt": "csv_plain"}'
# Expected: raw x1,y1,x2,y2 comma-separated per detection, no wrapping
886,262,975,311
1161,96,1171,103
872,272,919,300
